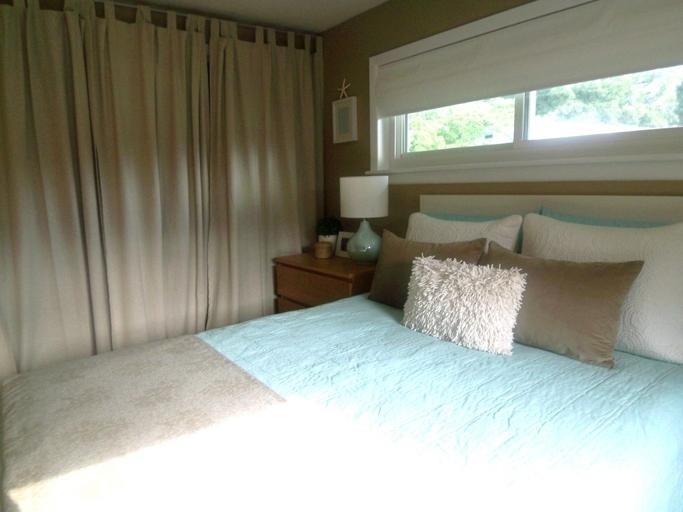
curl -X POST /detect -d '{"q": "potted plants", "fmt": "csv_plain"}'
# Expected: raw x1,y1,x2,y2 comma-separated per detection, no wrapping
315,217,343,252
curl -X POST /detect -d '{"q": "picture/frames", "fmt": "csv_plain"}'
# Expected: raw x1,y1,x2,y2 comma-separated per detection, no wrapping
331,95,358,144
335,231,354,259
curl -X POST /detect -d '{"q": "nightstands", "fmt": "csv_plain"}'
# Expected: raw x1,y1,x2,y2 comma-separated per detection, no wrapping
272,252,377,313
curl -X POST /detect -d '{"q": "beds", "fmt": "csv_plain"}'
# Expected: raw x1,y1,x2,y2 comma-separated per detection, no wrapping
0,195,683,508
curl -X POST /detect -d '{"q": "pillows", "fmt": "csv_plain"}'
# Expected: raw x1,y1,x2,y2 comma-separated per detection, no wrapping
407,211,522,257
478,239,646,368
519,211,681,366
367,228,489,311
401,252,529,359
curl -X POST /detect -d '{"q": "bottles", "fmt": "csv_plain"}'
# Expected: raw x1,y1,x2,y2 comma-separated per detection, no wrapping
314,241,334,260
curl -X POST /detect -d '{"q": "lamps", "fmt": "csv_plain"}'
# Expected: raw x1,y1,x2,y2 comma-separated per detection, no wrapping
339,175,389,266
